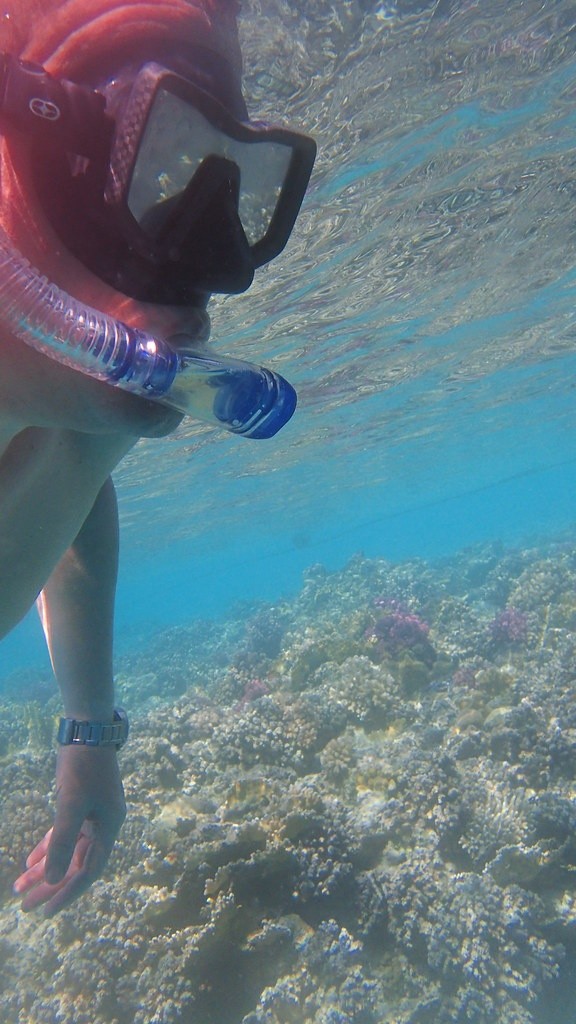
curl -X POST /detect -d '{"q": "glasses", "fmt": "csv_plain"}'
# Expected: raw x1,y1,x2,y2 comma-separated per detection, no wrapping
0,50,317,292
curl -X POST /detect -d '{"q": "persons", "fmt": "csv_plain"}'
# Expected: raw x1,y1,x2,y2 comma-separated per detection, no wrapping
0,0,318,919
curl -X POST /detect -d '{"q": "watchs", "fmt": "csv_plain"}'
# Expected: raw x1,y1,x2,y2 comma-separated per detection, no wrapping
57,708,130,752
